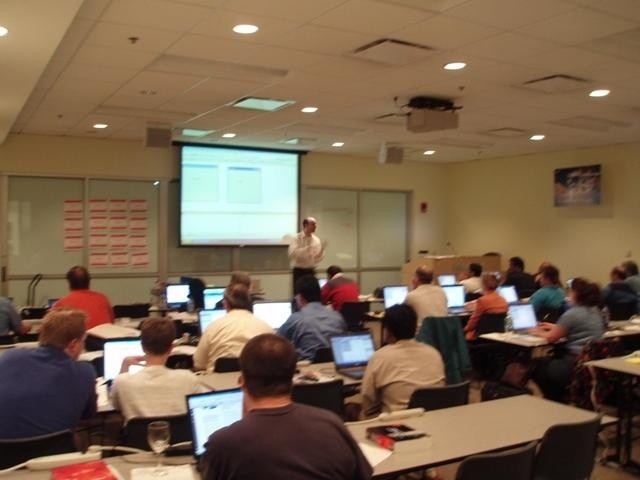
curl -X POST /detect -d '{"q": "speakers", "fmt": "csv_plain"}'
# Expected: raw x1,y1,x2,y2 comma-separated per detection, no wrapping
378,146,403,164
146,128,170,147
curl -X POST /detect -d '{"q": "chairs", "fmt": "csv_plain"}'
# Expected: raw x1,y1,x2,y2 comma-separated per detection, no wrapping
2,429,77,471
123,412,193,452
313,347,334,363
534,411,604,479
113,303,152,318
456,440,538,479
406,379,470,411
338,301,370,333
292,378,347,424
164,355,194,370
20,307,49,320
214,357,244,373
0,333,39,345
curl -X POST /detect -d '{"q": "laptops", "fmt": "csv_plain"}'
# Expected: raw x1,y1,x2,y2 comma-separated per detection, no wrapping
203,288,225,309
103,338,147,380
186,387,244,459
510,304,539,335
328,332,375,380
318,278,327,288
496,285,520,303
199,310,227,334
253,301,292,329
439,275,456,285
383,286,408,309
442,285,465,313
166,285,190,304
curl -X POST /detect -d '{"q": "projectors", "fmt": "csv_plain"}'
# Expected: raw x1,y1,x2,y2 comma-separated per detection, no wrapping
406,110,458,132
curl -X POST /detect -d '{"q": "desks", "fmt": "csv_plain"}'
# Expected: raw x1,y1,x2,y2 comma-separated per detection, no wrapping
366,311,386,347
342,394,620,477
95,362,365,411
401,254,500,291
479,319,640,348
583,350,640,478
0,453,200,479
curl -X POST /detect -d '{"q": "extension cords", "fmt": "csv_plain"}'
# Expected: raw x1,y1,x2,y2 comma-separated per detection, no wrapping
26,450,103,470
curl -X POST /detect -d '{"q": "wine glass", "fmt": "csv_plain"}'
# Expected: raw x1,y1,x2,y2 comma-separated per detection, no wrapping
146,419,172,479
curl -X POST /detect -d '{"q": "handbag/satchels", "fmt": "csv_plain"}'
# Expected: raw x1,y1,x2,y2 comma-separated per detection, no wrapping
501,362,531,389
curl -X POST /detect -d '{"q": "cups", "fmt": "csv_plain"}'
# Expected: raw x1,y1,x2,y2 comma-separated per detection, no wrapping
296,360,312,375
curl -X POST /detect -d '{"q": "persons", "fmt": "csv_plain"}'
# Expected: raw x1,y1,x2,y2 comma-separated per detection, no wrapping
288,215,326,278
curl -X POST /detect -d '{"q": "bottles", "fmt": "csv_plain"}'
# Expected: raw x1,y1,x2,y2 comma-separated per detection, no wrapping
600,305,610,327
504,311,513,339
